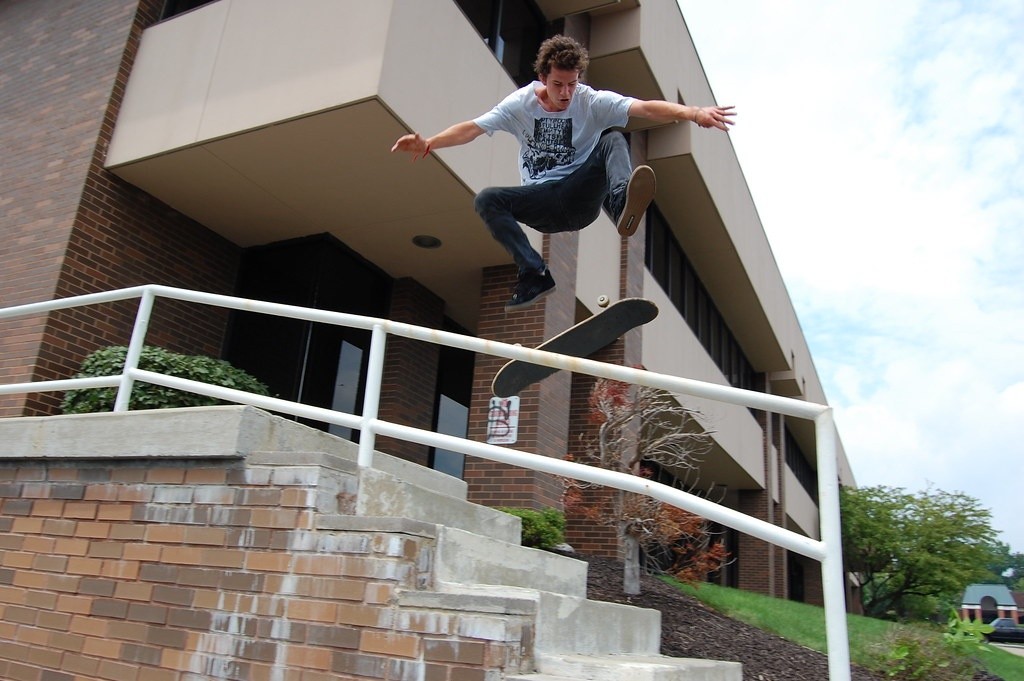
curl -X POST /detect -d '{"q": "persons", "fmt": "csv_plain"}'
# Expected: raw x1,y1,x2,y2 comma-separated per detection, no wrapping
390,35,738,312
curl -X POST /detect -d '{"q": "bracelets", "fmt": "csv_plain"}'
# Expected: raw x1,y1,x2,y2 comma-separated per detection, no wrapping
411,138,431,163
693,106,700,123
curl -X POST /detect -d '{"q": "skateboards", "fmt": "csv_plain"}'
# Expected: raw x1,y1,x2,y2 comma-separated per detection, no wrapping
491,295,659,398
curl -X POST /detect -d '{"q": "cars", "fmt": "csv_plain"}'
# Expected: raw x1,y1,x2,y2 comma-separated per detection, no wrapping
981,618,1024,644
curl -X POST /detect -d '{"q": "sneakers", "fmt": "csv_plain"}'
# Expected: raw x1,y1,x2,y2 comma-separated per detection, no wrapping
505,268,556,312
612,165,656,237
